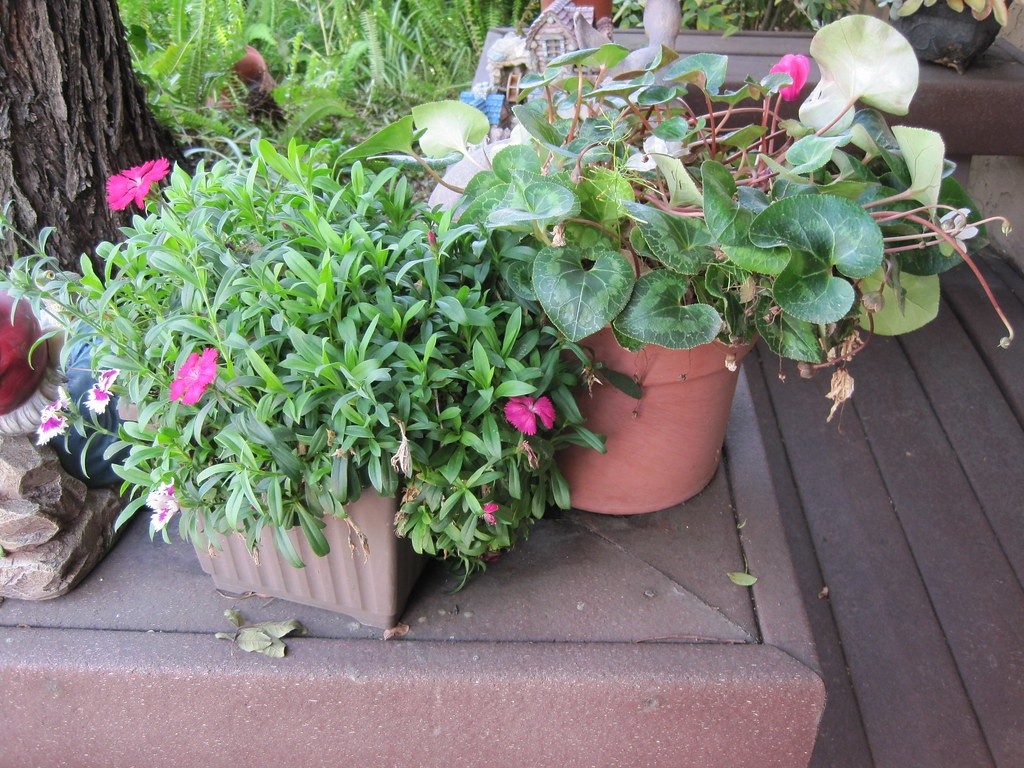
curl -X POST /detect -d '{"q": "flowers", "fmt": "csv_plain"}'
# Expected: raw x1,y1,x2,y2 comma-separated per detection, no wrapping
334,11,1017,381
1,134,642,592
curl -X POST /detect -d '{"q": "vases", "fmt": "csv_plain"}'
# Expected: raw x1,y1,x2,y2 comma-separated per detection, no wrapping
116,398,427,630
876,0,1014,75
555,324,761,516
